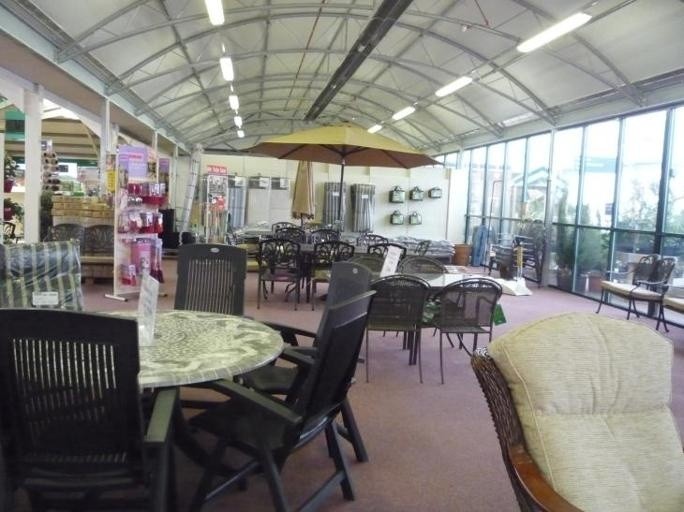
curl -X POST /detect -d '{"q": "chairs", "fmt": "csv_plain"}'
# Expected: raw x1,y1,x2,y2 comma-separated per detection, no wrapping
354,271,434,388
1,219,18,245
470,313,683,512
168,289,376,512
220,219,391,310
350,255,394,273
173,242,247,411
419,277,503,388
1,307,182,512
232,257,373,465
397,254,450,273
1,237,87,310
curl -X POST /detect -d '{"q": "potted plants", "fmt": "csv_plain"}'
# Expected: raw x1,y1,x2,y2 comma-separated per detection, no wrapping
552,188,605,294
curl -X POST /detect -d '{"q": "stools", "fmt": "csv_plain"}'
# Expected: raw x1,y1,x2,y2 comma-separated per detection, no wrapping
661,295,684,315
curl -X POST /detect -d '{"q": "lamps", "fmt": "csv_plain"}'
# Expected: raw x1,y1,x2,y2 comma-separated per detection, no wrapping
367,0,632,134
203,0,247,139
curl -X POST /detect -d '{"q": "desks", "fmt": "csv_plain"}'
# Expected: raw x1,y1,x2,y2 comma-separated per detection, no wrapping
2,309,287,491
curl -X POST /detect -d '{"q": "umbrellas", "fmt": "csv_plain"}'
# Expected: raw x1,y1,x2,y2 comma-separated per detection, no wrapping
293,158,316,227
239,120,445,239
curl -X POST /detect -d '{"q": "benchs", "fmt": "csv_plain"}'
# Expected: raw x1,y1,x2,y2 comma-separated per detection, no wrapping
41,222,115,284
594,254,676,334
